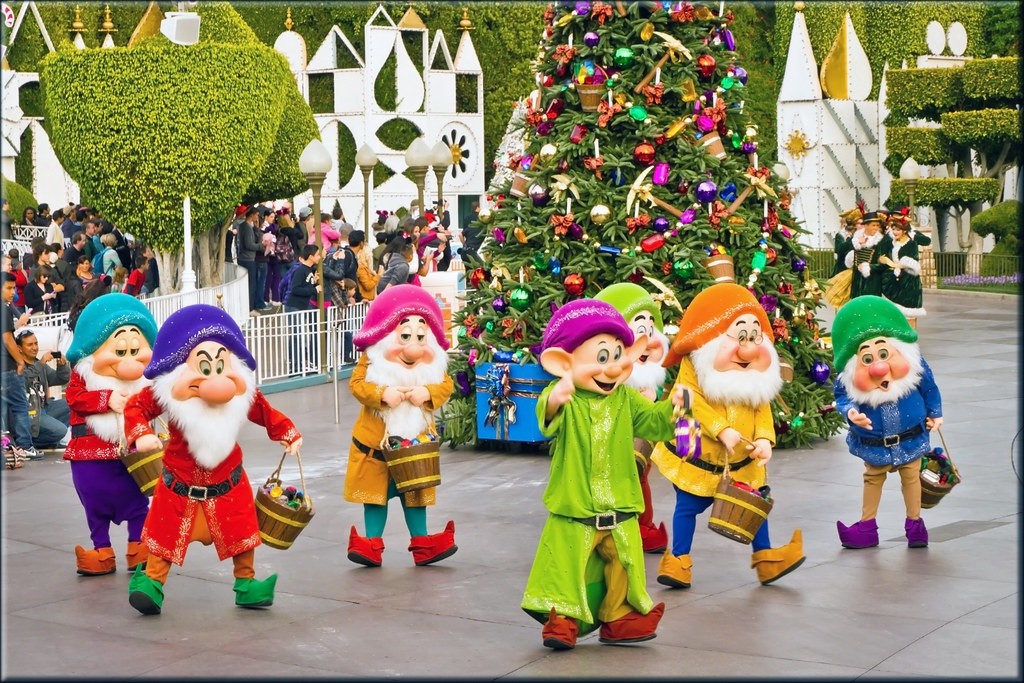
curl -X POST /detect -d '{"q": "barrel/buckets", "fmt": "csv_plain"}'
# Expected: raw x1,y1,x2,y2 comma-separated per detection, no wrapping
573,80,607,112
633,437,659,476
918,418,961,509
381,401,442,494
708,440,774,545
254,446,316,550
702,242,736,286
116,415,173,498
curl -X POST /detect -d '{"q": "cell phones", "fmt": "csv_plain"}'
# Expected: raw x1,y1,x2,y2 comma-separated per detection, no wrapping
51,352,61,358
26,308,33,314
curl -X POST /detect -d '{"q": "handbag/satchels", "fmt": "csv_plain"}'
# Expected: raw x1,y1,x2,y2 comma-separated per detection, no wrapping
1,443,26,470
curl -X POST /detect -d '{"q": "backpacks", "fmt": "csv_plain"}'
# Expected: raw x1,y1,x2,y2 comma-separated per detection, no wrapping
90,247,113,279
274,234,295,262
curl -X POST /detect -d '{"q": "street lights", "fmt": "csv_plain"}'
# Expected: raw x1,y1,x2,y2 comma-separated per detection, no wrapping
430,141,452,222
404,137,430,217
298,138,333,373
355,144,379,243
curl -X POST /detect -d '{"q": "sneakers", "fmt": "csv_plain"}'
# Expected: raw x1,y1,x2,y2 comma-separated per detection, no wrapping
25,446,45,458
38,443,68,453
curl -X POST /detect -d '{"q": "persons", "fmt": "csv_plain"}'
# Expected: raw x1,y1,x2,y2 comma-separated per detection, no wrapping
457,201,489,285
522,283,805,649
832,295,944,546
0,198,159,459
833,208,931,330
123,304,302,614
64,292,158,575
344,284,458,567
225,199,452,379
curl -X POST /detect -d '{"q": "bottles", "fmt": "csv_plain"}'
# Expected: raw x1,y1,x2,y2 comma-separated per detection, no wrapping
99,273,106,282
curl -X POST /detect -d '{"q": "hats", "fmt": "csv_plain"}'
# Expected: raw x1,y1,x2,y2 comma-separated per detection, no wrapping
299,206,313,218
888,206,907,217
857,200,881,224
234,205,253,221
